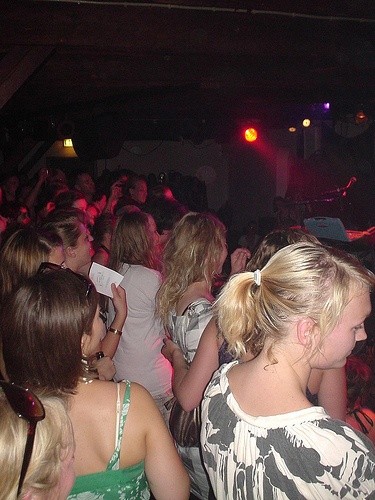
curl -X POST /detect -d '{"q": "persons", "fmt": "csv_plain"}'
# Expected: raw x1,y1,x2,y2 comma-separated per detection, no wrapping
199,241,375,500
156,211,252,500
0,377,77,500
0,260,191,500
0,165,375,447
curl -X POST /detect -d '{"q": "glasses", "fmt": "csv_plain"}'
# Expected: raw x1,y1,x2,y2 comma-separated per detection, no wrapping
0,380,45,500
36,262,92,297
16,211,30,219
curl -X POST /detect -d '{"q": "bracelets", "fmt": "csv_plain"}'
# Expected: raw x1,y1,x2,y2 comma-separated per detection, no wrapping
109,328,122,336
